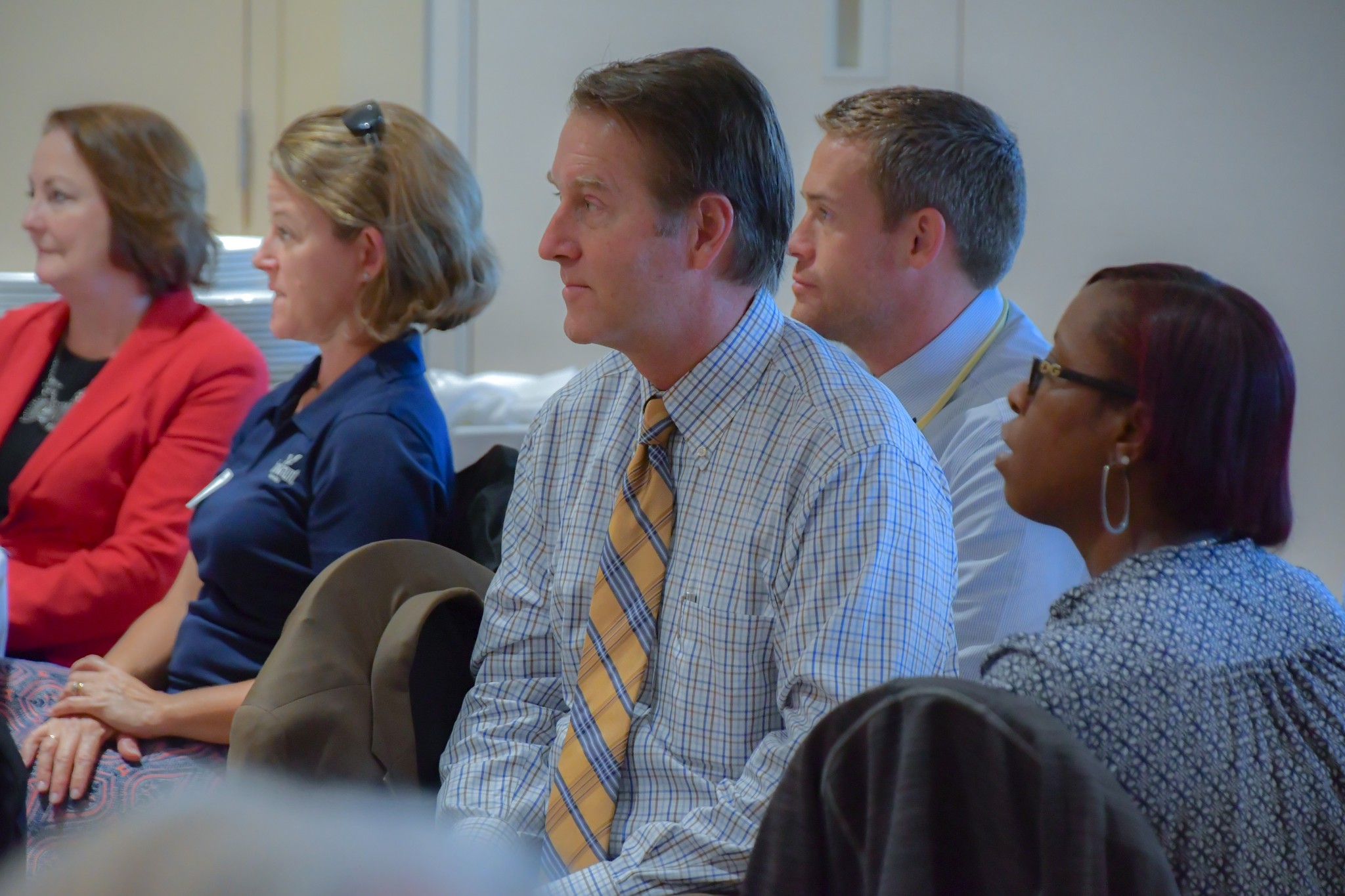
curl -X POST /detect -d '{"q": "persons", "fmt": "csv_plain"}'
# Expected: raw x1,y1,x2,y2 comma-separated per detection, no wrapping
0,100,271,670
976,262,1345,896
420,46,961,896
0,100,505,896
786,84,1091,683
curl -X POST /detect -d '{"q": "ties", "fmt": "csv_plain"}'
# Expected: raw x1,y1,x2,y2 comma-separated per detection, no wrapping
538,395,676,884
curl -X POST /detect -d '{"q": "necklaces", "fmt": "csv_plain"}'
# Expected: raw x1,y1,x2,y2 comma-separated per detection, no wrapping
38,338,89,426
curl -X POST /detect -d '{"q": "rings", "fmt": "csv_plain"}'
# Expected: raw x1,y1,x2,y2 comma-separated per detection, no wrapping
40,734,59,741
69,680,84,696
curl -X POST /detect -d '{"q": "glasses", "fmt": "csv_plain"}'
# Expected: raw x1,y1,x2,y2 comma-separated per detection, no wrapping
1025,357,1145,402
